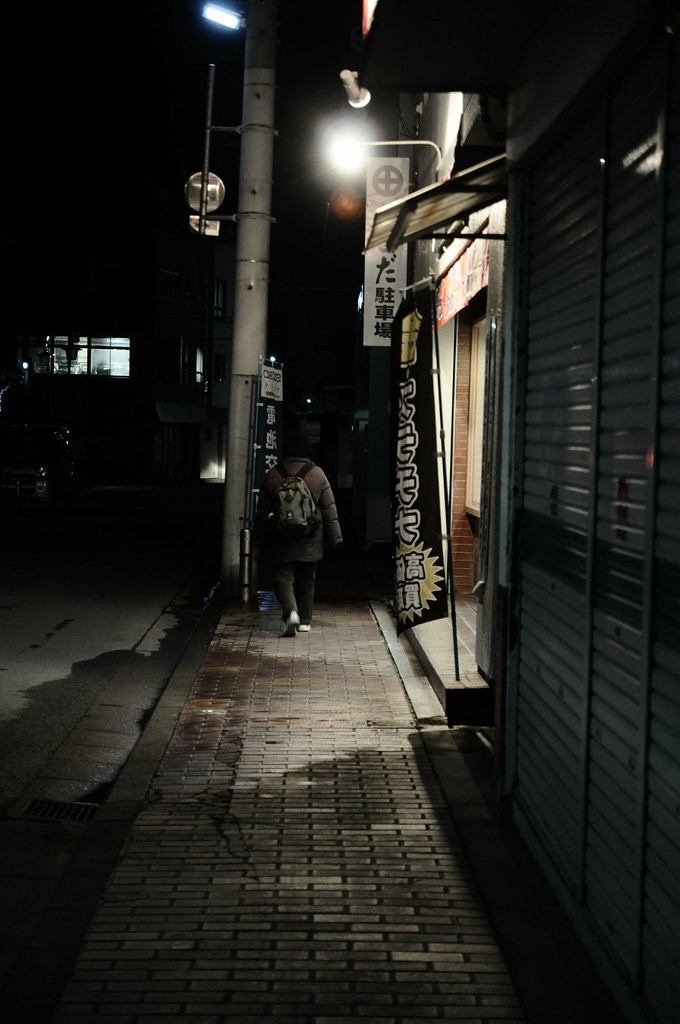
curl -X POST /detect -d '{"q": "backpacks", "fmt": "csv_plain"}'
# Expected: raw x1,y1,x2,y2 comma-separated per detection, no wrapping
274,461,317,541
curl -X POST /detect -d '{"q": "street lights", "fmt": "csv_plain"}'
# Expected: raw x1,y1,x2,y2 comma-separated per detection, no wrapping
194,0,272,608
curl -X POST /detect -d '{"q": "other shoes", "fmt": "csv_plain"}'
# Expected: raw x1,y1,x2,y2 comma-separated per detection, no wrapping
280,610,300,637
298,624,311,631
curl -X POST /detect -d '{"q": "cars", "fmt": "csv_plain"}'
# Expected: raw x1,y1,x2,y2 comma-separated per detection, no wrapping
0,422,81,512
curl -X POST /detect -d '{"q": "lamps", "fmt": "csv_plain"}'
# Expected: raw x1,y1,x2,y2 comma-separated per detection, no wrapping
340,68,370,109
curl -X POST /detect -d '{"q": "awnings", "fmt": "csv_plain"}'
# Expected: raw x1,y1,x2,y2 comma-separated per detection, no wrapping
360,155,510,250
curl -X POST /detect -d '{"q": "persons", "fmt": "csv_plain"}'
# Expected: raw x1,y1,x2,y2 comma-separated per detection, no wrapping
257,433,343,639
